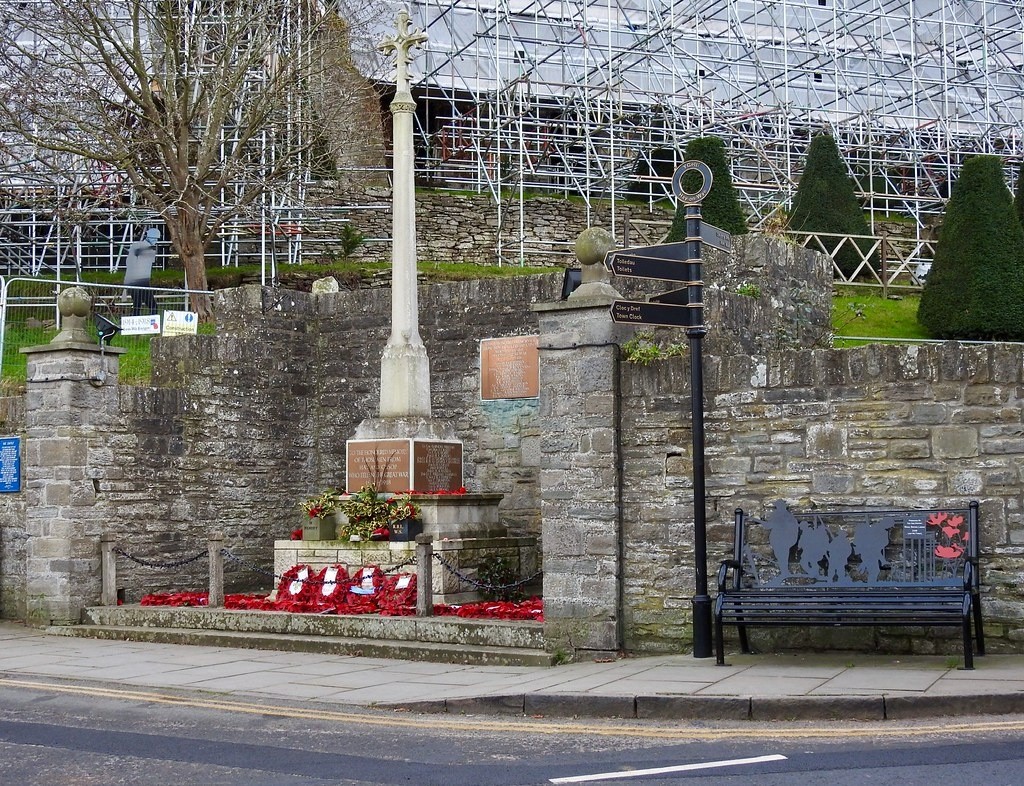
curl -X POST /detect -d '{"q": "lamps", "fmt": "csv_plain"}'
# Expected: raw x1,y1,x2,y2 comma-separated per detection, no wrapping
555,268,581,302
95,313,123,347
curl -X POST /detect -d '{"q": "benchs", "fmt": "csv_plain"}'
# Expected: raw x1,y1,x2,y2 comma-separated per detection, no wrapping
713,501,986,671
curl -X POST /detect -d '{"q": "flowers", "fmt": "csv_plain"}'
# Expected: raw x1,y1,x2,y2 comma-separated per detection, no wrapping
339,482,423,542
298,488,337,520
292,529,303,540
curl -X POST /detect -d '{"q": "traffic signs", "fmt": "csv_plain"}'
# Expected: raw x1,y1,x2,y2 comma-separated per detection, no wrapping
603,241,689,327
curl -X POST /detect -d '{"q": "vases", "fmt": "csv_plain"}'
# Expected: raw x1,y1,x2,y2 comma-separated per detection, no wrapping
303,517,336,541
389,520,423,542
